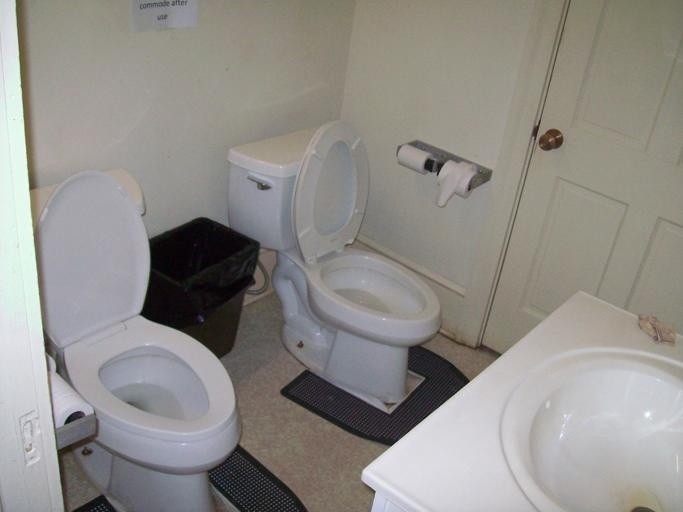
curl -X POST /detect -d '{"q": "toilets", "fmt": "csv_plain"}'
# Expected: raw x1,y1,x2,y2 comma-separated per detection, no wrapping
29,167,242,512
226,120,442,415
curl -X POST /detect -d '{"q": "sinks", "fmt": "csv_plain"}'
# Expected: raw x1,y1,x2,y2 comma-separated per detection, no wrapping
500,350,682,512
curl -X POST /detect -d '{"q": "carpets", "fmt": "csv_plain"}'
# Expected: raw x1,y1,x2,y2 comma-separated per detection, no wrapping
278,343,467,448
68,443,311,512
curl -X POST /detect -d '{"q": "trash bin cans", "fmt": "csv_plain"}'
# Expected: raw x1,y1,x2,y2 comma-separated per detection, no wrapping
141,217,259,359
